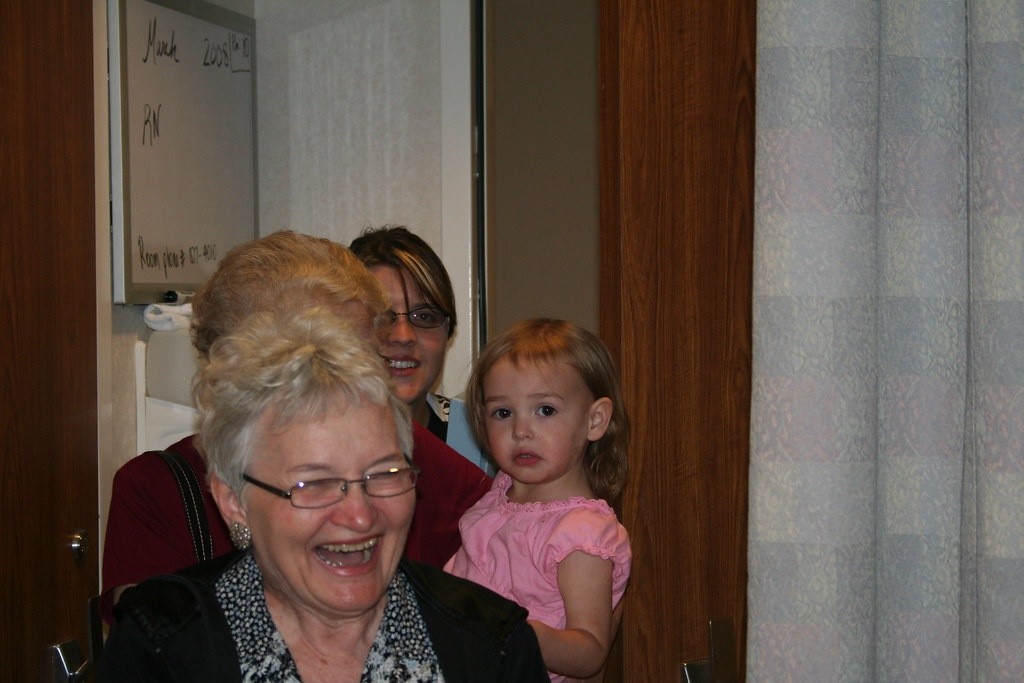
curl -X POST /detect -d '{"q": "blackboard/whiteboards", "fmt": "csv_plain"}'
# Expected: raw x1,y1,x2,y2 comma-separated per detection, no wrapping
110,0,261,310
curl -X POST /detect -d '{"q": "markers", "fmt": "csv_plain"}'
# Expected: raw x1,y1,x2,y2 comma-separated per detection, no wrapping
165,290,197,302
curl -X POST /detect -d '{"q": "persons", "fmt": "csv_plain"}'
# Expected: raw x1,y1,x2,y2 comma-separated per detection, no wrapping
346,226,495,476
103,231,495,623
443,317,634,683
93,300,549,683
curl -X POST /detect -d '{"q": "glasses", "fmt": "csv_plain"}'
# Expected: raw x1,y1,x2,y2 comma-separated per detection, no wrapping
242,453,421,509
389,306,450,328
